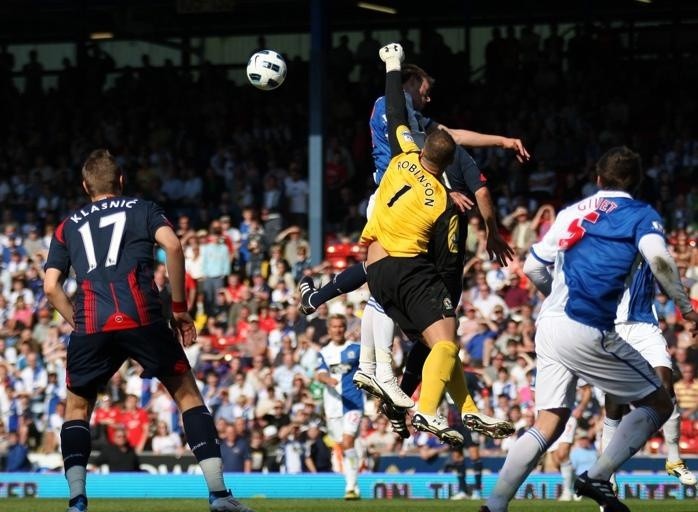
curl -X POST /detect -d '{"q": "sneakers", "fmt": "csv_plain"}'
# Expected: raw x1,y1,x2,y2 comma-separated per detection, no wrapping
574,472,630,511
68,497,86,511
344,490,360,500
298,276,318,314
209,488,250,511
353,368,515,447
664,459,697,486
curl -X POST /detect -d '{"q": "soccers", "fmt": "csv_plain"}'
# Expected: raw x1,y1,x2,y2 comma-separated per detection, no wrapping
247,49,287,90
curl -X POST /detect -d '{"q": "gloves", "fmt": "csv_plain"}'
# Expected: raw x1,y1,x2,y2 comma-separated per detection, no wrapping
379,41,405,73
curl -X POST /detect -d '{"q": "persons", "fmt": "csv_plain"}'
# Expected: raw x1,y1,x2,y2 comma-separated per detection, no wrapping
479,157,698,512
1,17,695,499
44,148,245,511
